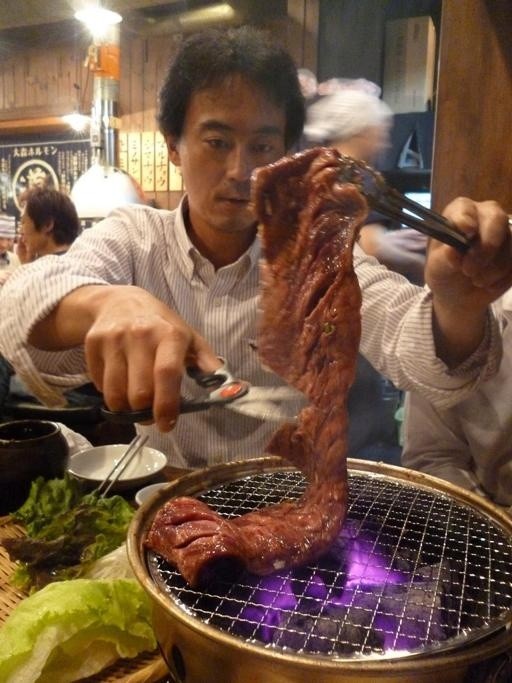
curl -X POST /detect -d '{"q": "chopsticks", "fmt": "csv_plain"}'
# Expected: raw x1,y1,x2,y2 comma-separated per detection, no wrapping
89,434,152,497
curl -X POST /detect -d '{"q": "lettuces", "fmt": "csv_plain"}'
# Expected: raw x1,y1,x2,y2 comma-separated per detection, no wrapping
0,477,158,683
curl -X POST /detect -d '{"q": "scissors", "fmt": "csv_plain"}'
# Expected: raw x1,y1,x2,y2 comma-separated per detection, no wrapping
97,356,307,428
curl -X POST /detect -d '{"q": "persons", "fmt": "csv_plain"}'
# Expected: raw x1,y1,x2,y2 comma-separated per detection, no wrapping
18,189,80,265
301,88,429,284
403,287,512,509
0,24,512,479
0,213,20,273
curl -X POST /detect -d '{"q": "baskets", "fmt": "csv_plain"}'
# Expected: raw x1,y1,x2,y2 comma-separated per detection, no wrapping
0,515,170,683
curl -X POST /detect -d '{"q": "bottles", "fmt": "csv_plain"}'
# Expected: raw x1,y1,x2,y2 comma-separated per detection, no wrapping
0,422,66,516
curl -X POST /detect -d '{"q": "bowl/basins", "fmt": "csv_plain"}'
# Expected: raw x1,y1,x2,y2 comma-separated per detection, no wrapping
66,445,168,493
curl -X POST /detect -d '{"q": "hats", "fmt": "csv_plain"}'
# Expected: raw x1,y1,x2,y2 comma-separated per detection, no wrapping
0,216,16,239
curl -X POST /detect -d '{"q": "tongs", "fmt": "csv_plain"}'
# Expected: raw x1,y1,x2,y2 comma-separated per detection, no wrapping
328,155,473,267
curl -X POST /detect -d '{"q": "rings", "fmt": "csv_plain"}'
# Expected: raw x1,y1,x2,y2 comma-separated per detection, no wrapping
506,217,512,225
